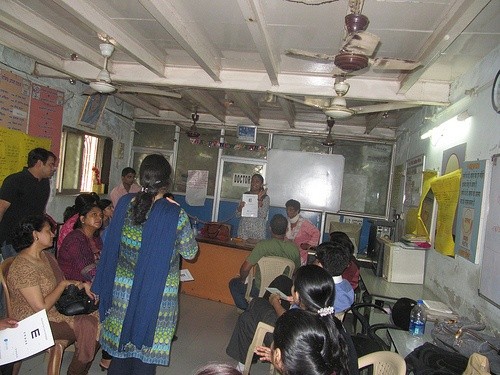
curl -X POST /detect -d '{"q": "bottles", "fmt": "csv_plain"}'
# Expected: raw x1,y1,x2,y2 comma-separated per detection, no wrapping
406,300,427,351
192,219,198,235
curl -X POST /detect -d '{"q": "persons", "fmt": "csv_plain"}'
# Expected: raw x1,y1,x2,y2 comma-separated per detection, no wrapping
264,231,361,309
229,213,301,308
164,193,173,200
7,215,100,375
56,192,115,372
254,264,358,375
199,362,242,375
286,200,320,268
226,241,355,371
0,317,18,375
110,166,141,207
90,154,197,375
270,309,333,375
0,148,57,315
234,174,270,240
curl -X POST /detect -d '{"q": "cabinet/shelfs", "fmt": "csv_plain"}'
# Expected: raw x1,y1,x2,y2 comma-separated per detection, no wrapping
382,242,425,285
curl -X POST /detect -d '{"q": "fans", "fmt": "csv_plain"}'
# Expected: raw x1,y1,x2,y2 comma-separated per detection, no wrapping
283,0,420,71
35,39,181,117
301,116,374,148
173,109,216,139
266,76,423,121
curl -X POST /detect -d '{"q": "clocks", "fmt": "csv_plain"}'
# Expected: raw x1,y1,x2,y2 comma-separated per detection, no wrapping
237,124,257,144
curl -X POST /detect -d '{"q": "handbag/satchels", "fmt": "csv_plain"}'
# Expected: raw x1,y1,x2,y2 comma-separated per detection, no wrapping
203,223,229,241
55,284,99,316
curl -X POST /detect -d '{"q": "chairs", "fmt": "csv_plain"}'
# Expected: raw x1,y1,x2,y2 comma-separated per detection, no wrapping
345,298,419,358
243,256,294,303
357,351,406,375
242,311,344,375
0,257,74,375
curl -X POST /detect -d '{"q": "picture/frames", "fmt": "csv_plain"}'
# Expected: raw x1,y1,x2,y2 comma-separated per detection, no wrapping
75,92,110,130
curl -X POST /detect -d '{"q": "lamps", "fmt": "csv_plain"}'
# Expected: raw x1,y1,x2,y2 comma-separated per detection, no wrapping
419,110,472,140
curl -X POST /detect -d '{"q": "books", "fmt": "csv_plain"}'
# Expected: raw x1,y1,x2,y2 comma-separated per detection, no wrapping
422,300,452,313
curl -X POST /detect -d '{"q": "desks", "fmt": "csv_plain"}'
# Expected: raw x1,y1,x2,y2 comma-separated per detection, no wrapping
179,234,255,307
307,248,378,265
359,266,448,338
388,329,433,358
